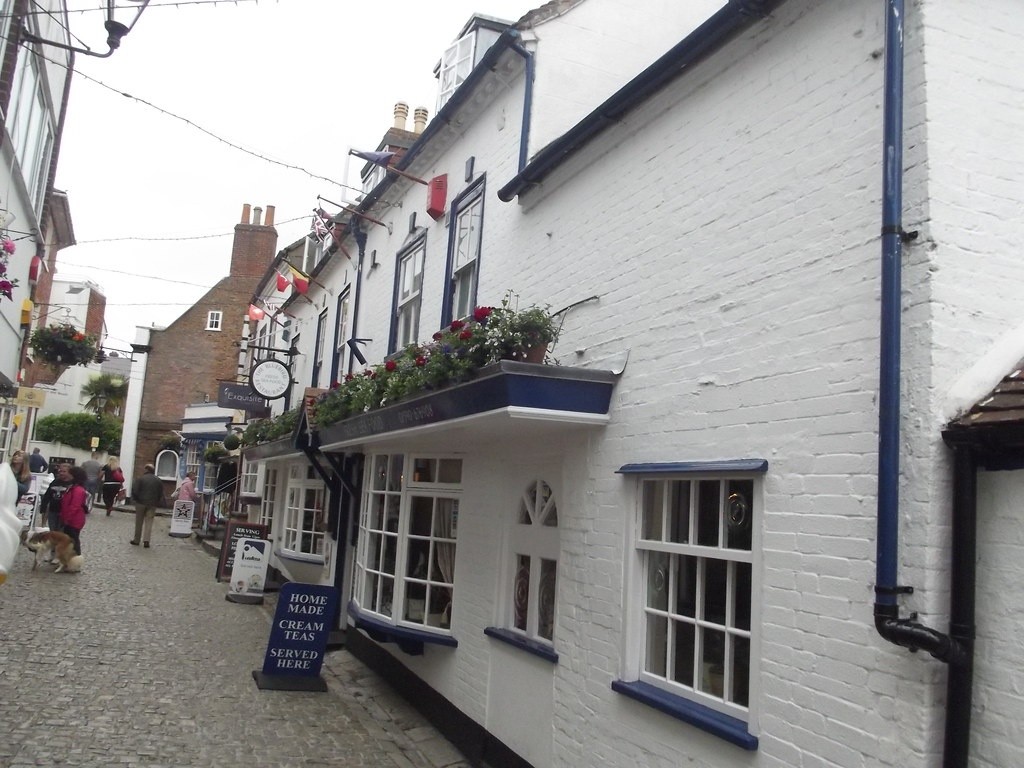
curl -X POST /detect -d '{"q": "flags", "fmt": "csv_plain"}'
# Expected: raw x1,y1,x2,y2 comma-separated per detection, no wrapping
249,303,265,321
288,264,310,295
277,273,290,292
309,150,396,242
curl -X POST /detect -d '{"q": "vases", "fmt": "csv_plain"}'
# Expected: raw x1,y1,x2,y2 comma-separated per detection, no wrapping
503,330,548,363
32,358,68,385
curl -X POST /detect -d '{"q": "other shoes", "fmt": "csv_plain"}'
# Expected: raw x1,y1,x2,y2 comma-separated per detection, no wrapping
130,540,138,545
144,541,149,547
107,509,110,515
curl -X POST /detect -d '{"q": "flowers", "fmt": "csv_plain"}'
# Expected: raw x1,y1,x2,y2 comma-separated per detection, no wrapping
380,342,431,408
425,320,473,386
348,364,384,414
325,373,355,420
29,322,98,368
309,391,343,428
0,238,19,302
468,288,564,367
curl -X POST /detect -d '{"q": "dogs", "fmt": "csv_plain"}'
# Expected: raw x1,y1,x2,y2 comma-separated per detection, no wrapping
20,528,74,573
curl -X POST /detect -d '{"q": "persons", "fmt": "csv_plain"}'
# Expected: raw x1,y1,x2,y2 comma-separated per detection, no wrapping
29,447,48,472
179,471,199,503
38,462,87,556
79,450,125,518
129,463,163,548
8,450,32,508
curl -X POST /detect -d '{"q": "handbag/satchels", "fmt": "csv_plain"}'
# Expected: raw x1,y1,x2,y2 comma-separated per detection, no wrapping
118,489,126,500
171,487,180,501
82,490,93,515
113,468,124,482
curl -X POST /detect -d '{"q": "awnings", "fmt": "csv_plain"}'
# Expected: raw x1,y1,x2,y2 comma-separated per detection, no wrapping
179,428,231,443
239,358,615,466
